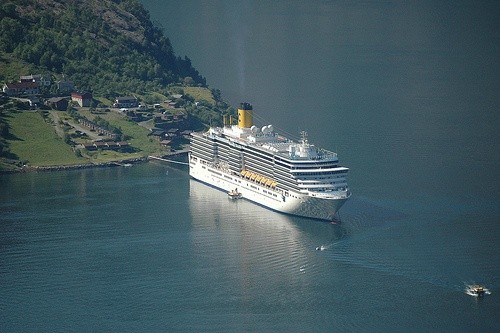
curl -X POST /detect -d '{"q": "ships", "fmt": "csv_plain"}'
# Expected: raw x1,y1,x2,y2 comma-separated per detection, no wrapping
188,101,352,222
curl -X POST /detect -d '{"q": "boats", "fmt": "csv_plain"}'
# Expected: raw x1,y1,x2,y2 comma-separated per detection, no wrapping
227,187,244,200
